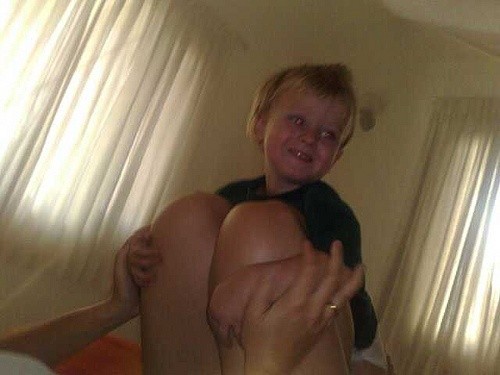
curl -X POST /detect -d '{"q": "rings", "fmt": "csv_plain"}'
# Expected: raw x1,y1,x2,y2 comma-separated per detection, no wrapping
328,301,340,314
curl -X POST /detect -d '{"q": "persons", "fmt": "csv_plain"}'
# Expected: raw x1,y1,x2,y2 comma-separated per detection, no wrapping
125,61,388,375
1,222,370,375
